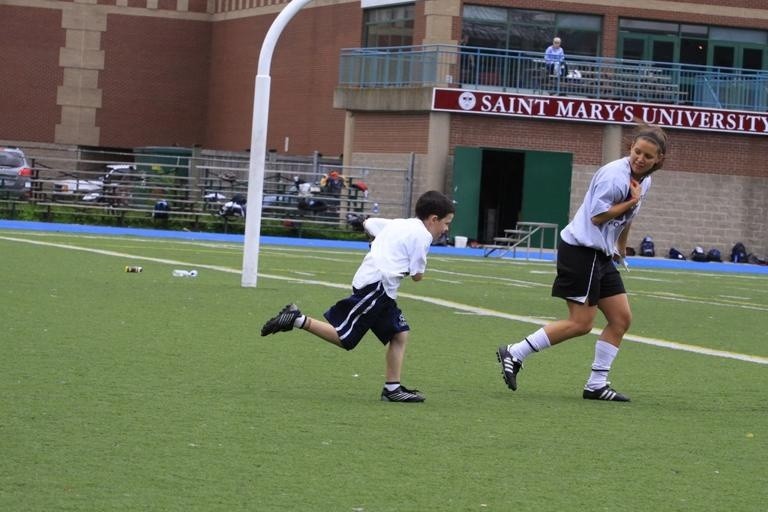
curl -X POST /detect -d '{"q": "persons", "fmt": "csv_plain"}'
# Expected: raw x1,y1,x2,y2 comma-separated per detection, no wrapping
496,123,668,403
260,188,454,404
543,36,568,79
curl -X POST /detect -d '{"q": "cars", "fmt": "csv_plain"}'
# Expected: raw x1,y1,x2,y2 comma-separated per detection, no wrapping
245,182,342,224
51,161,149,208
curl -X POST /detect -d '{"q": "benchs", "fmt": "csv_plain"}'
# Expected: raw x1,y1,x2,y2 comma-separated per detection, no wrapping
529,58,689,105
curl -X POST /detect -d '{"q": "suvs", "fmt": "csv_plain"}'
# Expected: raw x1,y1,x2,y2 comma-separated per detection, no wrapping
0,145,32,202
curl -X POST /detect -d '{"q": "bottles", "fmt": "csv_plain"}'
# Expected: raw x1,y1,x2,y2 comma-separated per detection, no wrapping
171,269,197,277
364,189,369,200
347,214,353,223
373,202,379,214
124,265,145,273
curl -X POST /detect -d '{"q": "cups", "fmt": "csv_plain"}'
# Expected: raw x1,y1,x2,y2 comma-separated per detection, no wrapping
294,175,299,184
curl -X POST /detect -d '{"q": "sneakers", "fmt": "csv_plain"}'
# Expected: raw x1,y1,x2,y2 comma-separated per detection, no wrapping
496,343,522,392
260,302,303,337
582,384,629,402
380,383,426,403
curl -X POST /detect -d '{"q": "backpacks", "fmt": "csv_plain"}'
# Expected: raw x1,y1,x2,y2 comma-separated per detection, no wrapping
624,236,768,266
